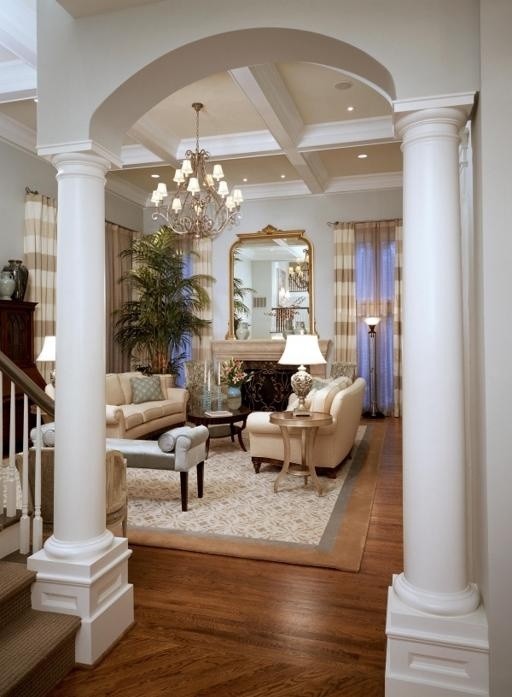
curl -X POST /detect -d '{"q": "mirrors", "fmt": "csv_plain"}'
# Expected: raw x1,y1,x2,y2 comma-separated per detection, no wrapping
228,225,317,340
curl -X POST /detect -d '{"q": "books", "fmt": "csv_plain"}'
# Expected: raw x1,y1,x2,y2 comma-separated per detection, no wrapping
204,409,234,418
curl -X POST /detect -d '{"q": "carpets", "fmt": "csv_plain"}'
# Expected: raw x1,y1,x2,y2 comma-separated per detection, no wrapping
2,414,387,574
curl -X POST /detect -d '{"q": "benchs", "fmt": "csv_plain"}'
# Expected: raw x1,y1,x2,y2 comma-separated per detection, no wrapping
29,422,211,511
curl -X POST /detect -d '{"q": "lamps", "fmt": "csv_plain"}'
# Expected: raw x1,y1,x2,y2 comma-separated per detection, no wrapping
362,317,386,419
34,334,58,362
288,247,309,292
148,103,244,238
277,333,328,417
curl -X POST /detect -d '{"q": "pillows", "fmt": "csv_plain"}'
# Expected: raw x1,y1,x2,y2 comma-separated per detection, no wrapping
43,427,55,446
158,426,191,452
285,377,328,413
128,376,165,404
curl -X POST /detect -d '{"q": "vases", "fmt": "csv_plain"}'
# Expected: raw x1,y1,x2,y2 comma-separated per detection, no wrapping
227,387,242,410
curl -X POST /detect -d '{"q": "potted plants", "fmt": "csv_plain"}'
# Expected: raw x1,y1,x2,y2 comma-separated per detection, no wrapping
110,224,215,389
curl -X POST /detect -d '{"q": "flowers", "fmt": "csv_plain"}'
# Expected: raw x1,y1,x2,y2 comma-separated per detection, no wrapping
218,359,253,388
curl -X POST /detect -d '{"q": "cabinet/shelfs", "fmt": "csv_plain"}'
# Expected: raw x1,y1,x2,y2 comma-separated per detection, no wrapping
1,300,38,399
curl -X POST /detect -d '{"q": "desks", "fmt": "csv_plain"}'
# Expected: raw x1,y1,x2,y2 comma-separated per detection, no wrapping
187,407,250,460
269,411,334,496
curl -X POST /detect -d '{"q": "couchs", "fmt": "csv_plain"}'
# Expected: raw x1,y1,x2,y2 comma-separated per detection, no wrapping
245,377,368,479
13,446,127,543
44,370,189,441
